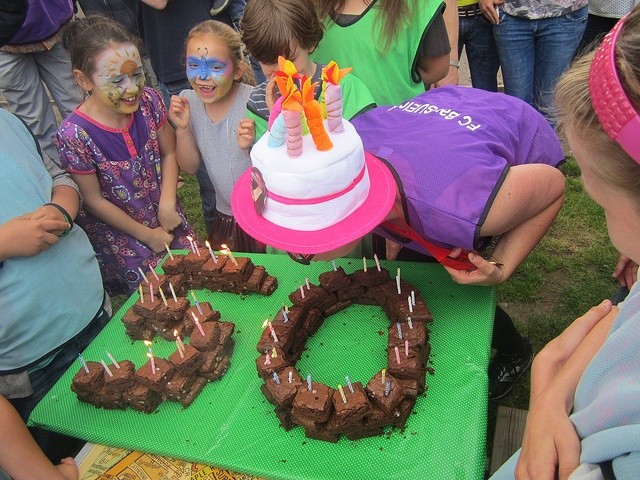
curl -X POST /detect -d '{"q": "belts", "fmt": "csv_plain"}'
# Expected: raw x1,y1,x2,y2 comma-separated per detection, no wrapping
456,8,483,18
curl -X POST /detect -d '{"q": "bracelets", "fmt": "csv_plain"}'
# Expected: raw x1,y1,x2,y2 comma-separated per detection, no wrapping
42,203,75,238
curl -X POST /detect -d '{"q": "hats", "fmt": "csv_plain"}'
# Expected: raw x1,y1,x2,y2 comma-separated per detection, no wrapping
230,56,397,253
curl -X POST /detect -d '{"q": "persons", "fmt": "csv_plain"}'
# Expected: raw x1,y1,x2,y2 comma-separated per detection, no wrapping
139,1,223,242
54,15,203,286
84,1,166,88
170,20,270,252
1,392,83,480
239,1,382,259
581,1,638,55
2,104,110,455
294,0,452,105
449,1,500,96
3,0,113,202
480,1,586,107
235,86,565,283
496,4,640,478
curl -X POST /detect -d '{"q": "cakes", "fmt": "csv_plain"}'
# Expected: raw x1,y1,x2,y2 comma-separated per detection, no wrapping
161,323,188,340
98,393,121,411
187,282,204,290
160,255,185,274
335,288,364,301
125,330,155,342
384,303,396,322
300,309,324,335
325,301,349,317
181,320,189,337
164,372,191,401
167,344,202,376
138,275,169,297
121,390,154,411
270,306,305,329
291,344,303,360
326,412,363,434
202,346,225,372
70,384,99,411
315,295,334,309
260,382,275,403
388,322,426,347
202,254,229,272
255,325,296,353
396,299,433,322
288,283,326,310
133,293,160,316
367,408,400,429
133,356,173,391
263,365,303,408
202,356,230,381
385,281,417,301
219,321,234,346
386,345,419,380
332,381,372,427
157,298,188,319
222,258,254,282
291,380,334,423
162,273,185,293
207,277,233,292
399,379,418,398
390,399,414,429
344,427,385,441
271,406,297,432
289,410,318,432
356,293,380,306
235,264,268,292
417,368,425,394
259,277,276,296
369,282,384,302
255,349,287,377
173,285,187,297
185,302,223,326
188,321,218,349
291,329,305,354
121,307,145,330
73,361,103,391
190,272,207,281
317,267,351,292
103,360,135,392
353,265,389,288
183,248,209,272
420,341,430,364
303,426,337,443
366,369,404,412
178,378,207,406
146,316,171,334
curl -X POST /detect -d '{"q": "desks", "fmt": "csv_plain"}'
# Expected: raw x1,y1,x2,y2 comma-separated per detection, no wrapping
26,248,496,480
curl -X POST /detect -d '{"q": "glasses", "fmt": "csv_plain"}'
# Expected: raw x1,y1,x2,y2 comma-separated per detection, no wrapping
286,252,315,266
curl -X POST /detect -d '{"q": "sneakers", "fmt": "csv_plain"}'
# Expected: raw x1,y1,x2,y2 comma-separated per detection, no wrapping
487,338,534,401
